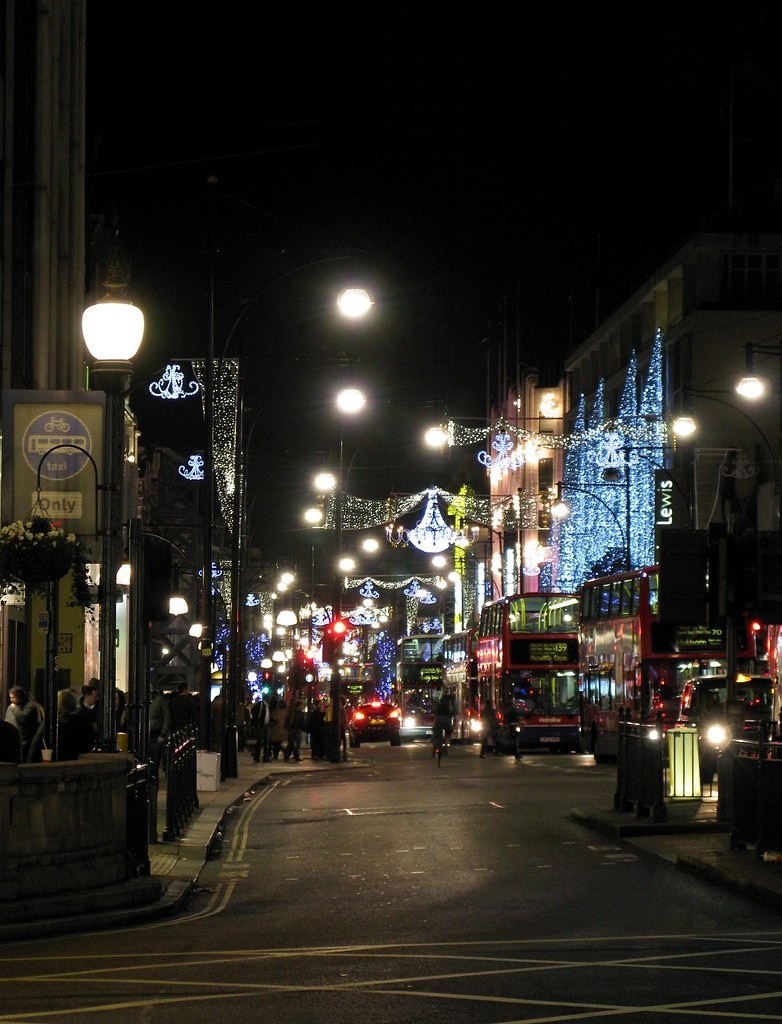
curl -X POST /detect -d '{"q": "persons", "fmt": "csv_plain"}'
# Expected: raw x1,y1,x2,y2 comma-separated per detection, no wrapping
58,678,129,760
433,698,456,745
150,682,348,793
480,700,523,758
0,685,47,764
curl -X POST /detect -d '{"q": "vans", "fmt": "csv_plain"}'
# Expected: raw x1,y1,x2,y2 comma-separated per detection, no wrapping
676,672,776,783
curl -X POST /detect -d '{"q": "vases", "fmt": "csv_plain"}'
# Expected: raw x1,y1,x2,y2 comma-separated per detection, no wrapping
7,551,72,583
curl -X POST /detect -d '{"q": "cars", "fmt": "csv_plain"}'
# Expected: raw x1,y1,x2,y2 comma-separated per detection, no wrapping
347,702,402,747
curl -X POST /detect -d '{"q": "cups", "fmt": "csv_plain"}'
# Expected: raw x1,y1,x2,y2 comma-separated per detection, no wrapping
41,750,54,762
117,733,128,751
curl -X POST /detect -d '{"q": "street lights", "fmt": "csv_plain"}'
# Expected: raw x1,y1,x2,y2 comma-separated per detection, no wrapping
78,251,146,757
551,480,631,570
233,566,300,752
197,276,376,782
302,505,327,651
334,388,364,762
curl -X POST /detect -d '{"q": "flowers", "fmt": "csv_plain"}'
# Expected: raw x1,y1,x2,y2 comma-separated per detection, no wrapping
0,518,100,626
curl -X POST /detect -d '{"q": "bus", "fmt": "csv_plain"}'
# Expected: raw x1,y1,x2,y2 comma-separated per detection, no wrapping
476,591,586,754
338,659,377,727
395,634,448,739
440,627,483,744
575,564,760,764
